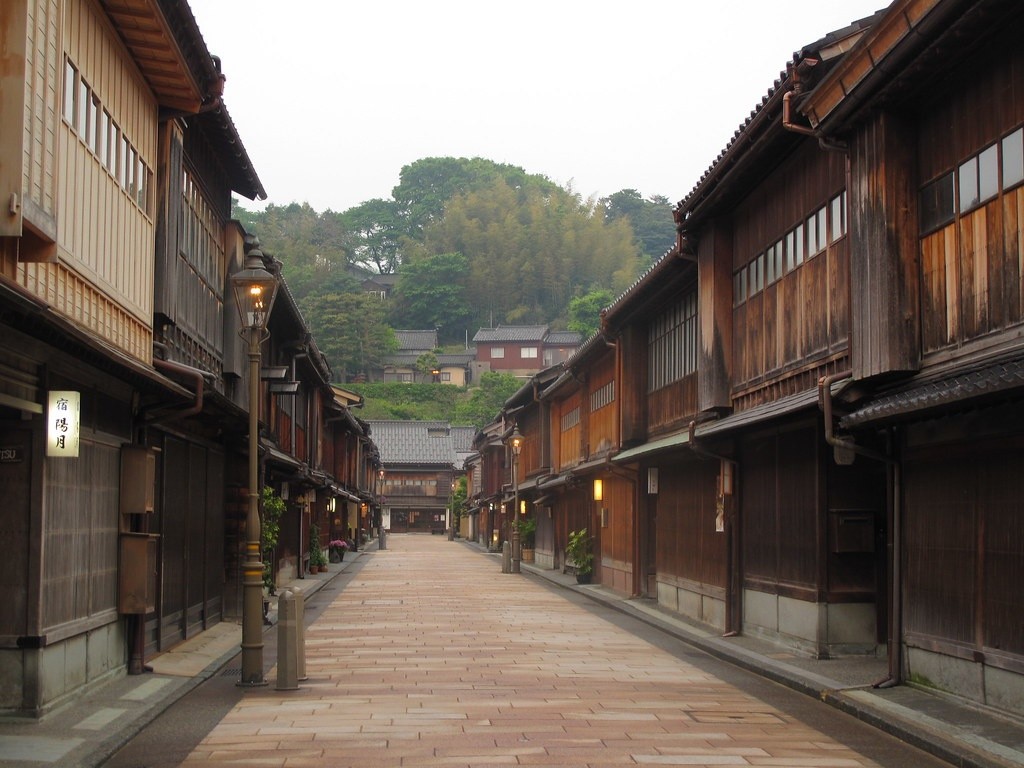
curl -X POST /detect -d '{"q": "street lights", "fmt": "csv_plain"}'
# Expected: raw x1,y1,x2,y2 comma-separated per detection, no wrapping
507,422,525,574
377,467,385,510
450,481,456,540
230,234,281,687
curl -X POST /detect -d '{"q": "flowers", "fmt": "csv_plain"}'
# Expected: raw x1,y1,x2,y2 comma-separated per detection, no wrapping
329,540,350,550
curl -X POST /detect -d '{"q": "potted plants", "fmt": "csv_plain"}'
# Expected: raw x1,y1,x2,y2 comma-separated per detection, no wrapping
564,528,597,584
512,518,535,560
308,524,329,574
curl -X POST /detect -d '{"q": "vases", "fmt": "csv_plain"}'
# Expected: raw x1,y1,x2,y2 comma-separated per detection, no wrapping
328,548,344,562
